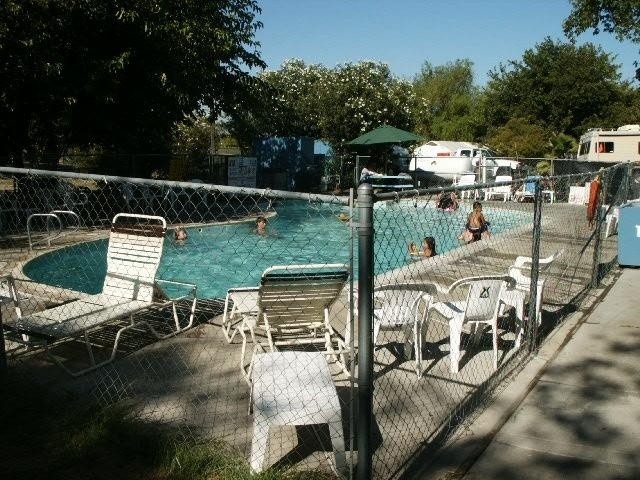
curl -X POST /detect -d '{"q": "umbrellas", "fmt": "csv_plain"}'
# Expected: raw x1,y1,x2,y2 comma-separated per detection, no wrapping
345,123,427,173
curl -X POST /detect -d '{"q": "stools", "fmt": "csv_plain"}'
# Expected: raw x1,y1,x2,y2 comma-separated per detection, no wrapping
541,190,557,204
249,351,346,477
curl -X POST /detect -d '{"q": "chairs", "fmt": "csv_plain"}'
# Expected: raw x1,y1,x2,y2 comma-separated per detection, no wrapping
221,264,350,382
498,250,569,350
345,282,437,381
422,276,518,374
2,210,207,377
450,173,543,205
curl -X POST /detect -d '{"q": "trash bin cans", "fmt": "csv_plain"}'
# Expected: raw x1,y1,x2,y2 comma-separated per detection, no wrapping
618,202,640,266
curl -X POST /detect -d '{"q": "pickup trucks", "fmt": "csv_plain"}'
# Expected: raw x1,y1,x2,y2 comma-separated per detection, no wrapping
410,146,524,180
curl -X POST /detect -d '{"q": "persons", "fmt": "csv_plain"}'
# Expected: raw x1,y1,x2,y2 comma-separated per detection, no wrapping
250,217,266,236
408,237,437,257
174,227,187,240
438,189,459,211
459,202,491,245
